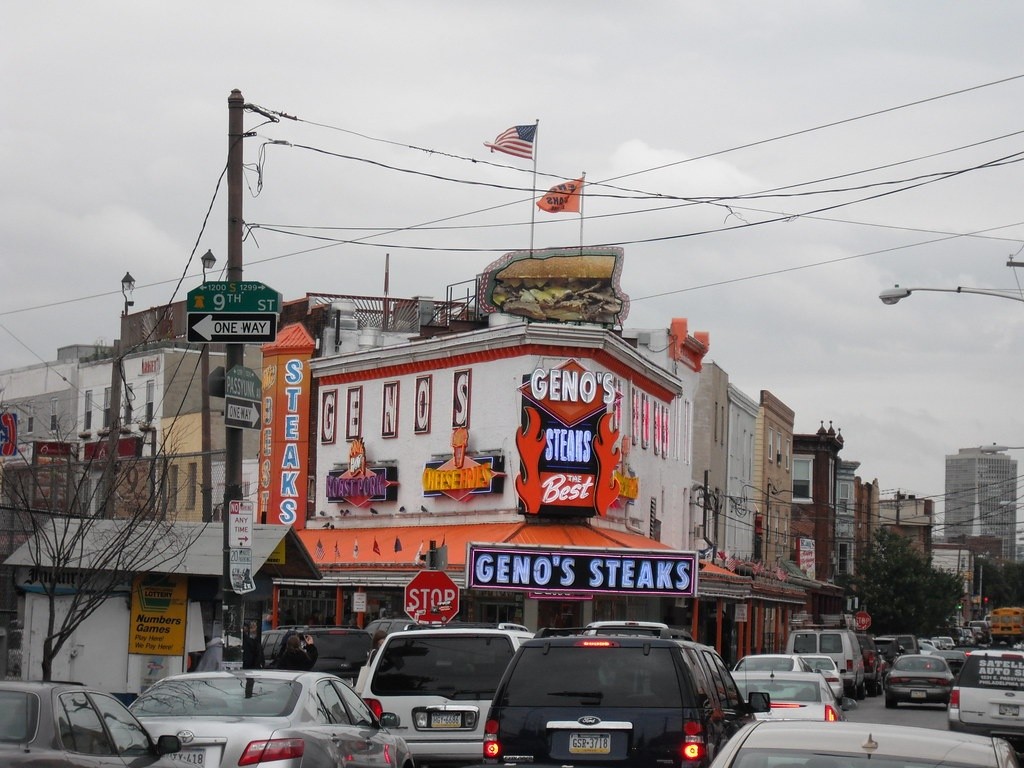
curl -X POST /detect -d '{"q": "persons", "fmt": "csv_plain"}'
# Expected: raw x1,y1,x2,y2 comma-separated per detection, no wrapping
305,609,336,628
281,634,318,671
362,630,387,667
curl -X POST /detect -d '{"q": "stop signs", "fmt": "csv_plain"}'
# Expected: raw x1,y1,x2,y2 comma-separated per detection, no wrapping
404,570,460,627
853,611,871,630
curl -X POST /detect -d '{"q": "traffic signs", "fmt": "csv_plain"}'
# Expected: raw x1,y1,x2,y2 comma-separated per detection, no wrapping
227,500,253,548
223,395,262,430
186,312,277,343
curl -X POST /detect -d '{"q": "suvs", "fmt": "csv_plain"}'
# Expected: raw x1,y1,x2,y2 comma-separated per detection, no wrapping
483,624,770,768
0,679,187,768
947,649,1024,751
858,635,900,698
362,621,534,768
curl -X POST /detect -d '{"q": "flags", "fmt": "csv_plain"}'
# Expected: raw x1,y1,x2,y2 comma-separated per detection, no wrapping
536,177,583,215
314,536,423,564
483,124,537,162
726,553,788,580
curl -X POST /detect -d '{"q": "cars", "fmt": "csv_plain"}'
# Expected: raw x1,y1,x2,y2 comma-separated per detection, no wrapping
730,653,847,722
709,716,1021,768
126,669,414,768
884,628,985,707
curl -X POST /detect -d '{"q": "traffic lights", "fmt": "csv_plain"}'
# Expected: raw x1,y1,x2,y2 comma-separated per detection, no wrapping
981,596,987,602
957,602,962,609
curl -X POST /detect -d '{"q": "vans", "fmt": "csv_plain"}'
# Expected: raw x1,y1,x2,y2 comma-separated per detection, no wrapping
785,628,866,700
894,634,923,655
966,621,987,631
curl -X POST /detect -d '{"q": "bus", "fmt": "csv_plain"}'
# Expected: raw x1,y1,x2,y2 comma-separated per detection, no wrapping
988,607,1024,646
